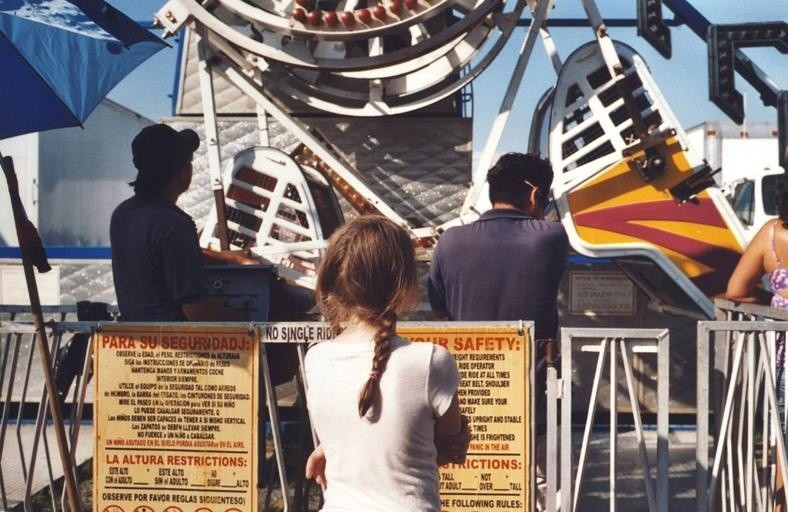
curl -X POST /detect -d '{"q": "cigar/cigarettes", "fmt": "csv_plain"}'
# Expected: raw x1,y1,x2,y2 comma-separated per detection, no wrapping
525,179,543,197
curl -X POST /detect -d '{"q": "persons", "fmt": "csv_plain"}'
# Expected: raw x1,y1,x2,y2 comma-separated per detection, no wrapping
427,152,569,435
304,214,469,512
109,124,262,321
724,171,788,512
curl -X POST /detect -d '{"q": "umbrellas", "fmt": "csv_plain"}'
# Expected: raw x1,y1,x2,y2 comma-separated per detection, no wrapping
0,0,173,141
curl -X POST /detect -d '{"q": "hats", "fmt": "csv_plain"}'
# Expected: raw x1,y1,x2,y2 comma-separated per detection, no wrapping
129,121,202,171
